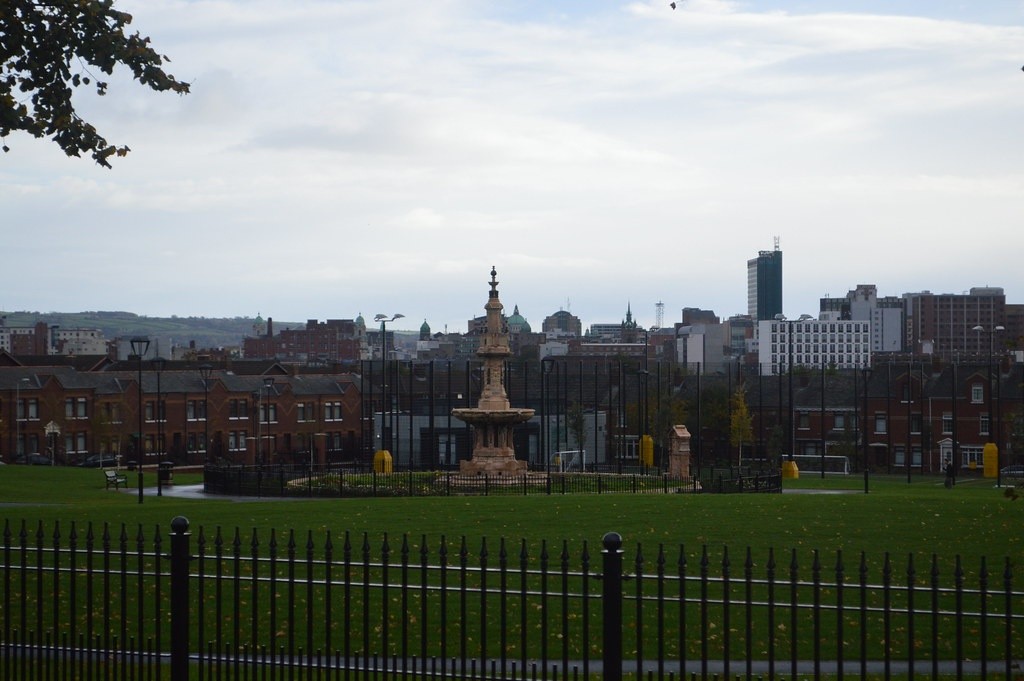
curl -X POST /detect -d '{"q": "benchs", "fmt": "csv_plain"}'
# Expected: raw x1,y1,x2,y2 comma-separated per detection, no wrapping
103,469,128,489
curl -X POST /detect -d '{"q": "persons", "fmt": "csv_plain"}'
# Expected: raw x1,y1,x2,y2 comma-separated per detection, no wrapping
942,459,956,486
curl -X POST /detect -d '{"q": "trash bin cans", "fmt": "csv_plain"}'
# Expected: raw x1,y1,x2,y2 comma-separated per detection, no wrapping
127,461,136,471
160,462,174,485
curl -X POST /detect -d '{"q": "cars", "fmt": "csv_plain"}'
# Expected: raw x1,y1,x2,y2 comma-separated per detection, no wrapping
999,464,1024,477
77,453,118,467
14,452,50,465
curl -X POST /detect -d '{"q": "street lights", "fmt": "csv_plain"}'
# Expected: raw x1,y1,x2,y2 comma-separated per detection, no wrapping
262,376,275,477
130,335,151,503
635,369,649,439
635,324,661,469
150,356,165,496
972,326,1005,478
16,377,31,456
861,366,873,494
198,363,213,468
373,312,405,477
539,355,556,495
774,313,813,478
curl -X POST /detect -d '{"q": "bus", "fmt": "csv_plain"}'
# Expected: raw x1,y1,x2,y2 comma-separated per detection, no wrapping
781,454,852,475
